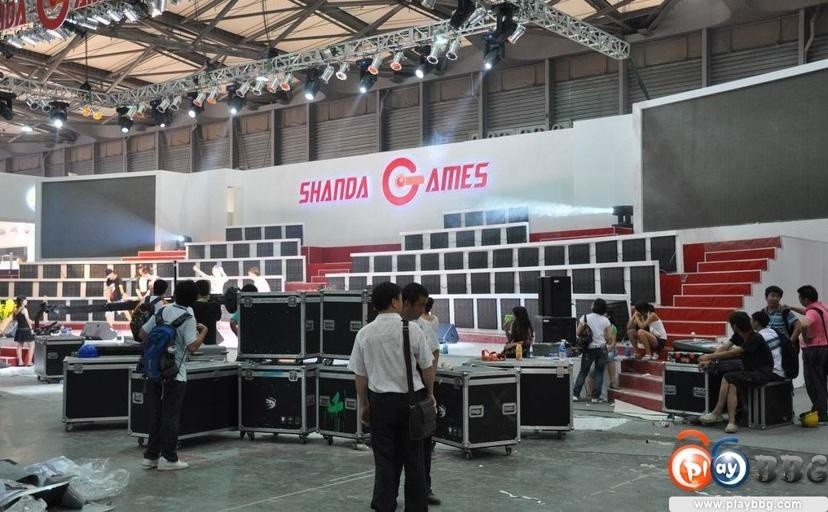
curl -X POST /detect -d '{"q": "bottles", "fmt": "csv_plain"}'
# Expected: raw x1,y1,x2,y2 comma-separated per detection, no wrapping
61,326,72,336
504,315,513,321
625,342,631,356
440,361,453,370
515,343,523,359
558,342,567,361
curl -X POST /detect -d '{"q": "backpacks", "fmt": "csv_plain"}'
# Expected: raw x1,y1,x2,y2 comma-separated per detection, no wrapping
142,305,194,400
130,295,165,342
773,328,799,378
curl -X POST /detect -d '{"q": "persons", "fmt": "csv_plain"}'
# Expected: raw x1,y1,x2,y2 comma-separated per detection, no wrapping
572,298,611,404
750,311,785,382
345,281,438,512
136,280,208,471
500,306,534,358
409,296,438,331
1,258,272,370
759,286,802,351
626,302,667,361
696,312,773,432
781,285,827,422
399,282,443,505
597,309,620,390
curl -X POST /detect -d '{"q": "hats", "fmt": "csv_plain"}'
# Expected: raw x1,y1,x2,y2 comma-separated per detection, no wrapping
104,269,112,275
78,345,98,358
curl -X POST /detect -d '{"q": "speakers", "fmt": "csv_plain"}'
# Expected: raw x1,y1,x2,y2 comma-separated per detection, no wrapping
437,324,460,344
605,301,629,342
80,322,117,340
535,315,576,344
537,276,571,317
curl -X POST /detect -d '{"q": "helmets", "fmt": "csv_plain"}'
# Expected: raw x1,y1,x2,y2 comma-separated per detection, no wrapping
799,411,819,427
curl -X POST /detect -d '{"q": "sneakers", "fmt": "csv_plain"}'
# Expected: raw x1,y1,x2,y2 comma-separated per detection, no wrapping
157,456,189,471
427,494,441,506
630,353,641,359
141,457,158,470
591,398,603,403
641,354,652,361
725,423,737,433
573,396,580,402
698,413,724,423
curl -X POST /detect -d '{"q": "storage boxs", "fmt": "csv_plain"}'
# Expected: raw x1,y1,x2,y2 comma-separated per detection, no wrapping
34,289,574,458
660,338,793,428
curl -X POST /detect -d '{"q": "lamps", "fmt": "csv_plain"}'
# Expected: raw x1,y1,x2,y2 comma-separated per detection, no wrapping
176,234,192,250
611,205,634,228
0,0,528,132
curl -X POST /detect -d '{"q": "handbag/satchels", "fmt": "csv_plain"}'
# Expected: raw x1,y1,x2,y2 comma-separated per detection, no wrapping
576,314,593,352
403,320,436,441
